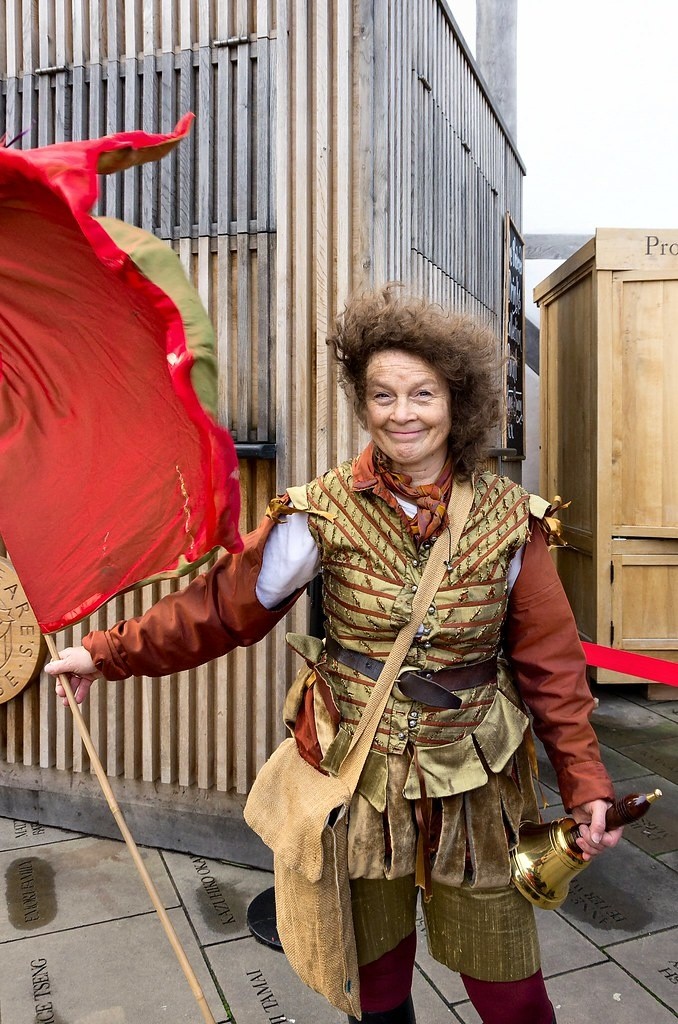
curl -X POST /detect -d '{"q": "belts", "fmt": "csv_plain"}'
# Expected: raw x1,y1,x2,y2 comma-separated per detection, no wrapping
323,640,499,708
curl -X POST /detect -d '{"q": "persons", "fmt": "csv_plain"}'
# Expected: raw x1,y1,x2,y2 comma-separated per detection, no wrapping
45,292,624,1024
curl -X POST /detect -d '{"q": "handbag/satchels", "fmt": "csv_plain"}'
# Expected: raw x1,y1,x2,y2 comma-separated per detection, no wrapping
244,737,362,1017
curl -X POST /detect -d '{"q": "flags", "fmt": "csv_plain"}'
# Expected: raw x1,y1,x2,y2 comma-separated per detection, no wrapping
0,113,246,635
